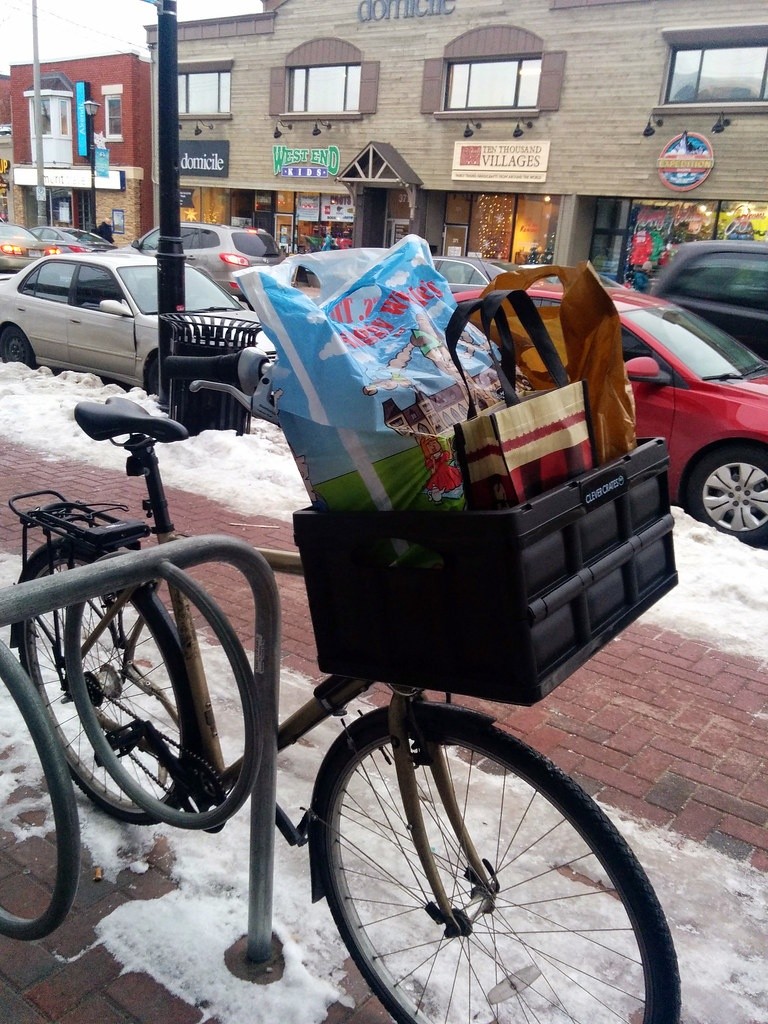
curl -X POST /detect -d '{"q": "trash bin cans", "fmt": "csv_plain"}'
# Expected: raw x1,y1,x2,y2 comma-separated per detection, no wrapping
159,310,264,441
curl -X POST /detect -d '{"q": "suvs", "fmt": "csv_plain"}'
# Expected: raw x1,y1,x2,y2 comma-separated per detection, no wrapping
103,221,288,311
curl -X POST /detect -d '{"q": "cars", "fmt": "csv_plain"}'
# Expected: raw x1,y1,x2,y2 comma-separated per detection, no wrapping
27,226,118,255
0,251,278,412
429,255,637,296
0,222,64,273
426,283,767,549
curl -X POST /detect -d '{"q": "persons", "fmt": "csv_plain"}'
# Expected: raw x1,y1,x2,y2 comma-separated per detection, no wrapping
99,217,115,244
633,262,652,295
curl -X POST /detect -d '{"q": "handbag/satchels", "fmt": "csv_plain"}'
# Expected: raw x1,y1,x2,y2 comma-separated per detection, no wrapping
445,288,598,509
231,234,535,513
469,259,638,465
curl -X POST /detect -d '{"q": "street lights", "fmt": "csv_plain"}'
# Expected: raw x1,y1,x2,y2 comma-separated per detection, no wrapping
83,97,102,235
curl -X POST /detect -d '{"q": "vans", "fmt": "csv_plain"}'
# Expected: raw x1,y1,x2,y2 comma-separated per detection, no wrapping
646,238,767,366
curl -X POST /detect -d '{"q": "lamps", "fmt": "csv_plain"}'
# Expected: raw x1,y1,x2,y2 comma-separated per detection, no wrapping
711,111,732,135
464,118,481,137
312,119,331,136
644,113,664,135
274,119,292,139
194,118,212,136
512,117,533,136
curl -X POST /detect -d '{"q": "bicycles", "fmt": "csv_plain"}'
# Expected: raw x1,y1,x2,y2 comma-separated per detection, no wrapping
7,346,682,1023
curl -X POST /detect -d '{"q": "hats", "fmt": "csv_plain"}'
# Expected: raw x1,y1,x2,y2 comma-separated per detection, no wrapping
642,260,652,270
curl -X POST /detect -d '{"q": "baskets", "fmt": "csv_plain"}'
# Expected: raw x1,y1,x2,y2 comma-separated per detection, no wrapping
292,436,679,707
